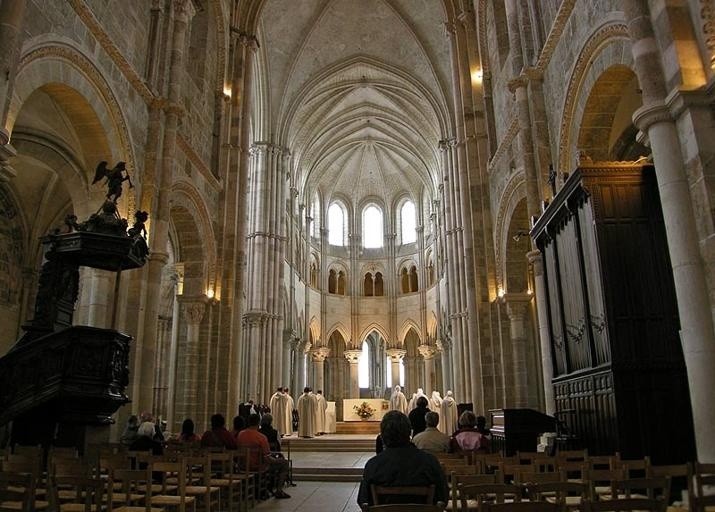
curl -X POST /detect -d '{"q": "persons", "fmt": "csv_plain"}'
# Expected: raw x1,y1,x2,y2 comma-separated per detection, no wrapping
270,386,328,438
119,401,291,500
91,160,135,204
357,386,494,510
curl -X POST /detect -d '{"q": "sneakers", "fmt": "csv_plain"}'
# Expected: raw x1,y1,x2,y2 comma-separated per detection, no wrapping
275,490,292,497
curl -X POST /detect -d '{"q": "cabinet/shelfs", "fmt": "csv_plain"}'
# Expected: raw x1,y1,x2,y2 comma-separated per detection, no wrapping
488,408,554,484
529,150,696,506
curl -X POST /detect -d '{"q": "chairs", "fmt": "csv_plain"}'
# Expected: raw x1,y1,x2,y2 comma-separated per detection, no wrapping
0,440,297,512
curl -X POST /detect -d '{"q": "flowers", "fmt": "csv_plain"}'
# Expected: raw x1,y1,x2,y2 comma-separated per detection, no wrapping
352,402,376,419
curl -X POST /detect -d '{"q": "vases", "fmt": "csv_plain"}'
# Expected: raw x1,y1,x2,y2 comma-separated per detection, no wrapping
361,417,368,421
